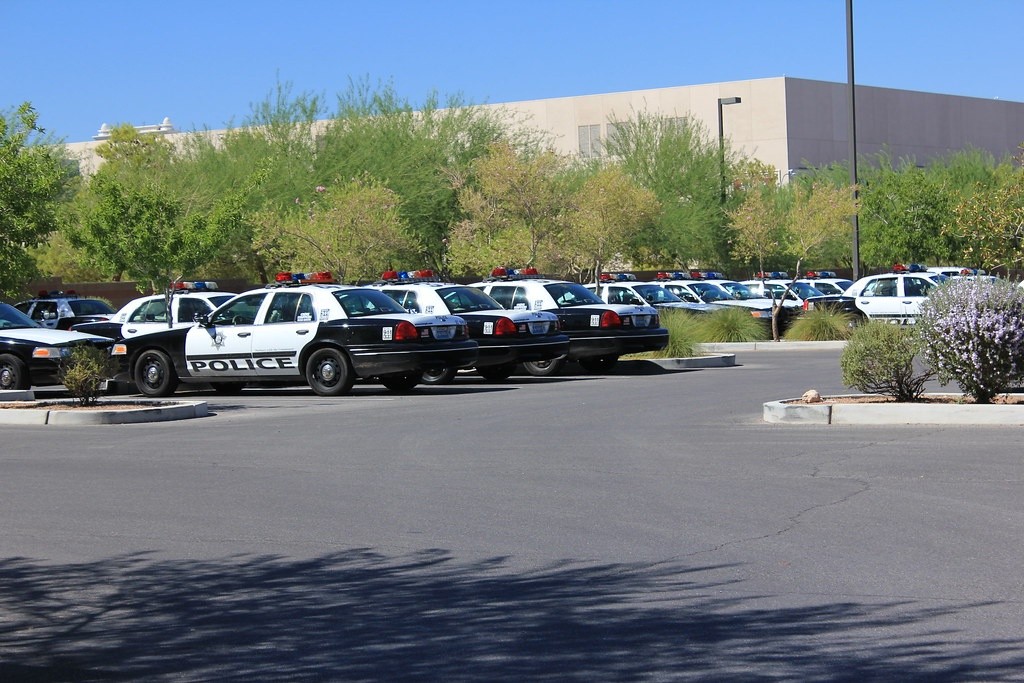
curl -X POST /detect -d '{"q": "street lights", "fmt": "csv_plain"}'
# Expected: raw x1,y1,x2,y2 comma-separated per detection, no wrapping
715,98,745,206
844,1,866,278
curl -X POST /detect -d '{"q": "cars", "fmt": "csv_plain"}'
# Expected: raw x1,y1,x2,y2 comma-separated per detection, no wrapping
0,290,117,392
802,266,1008,336
54,267,669,398
573,266,857,333
